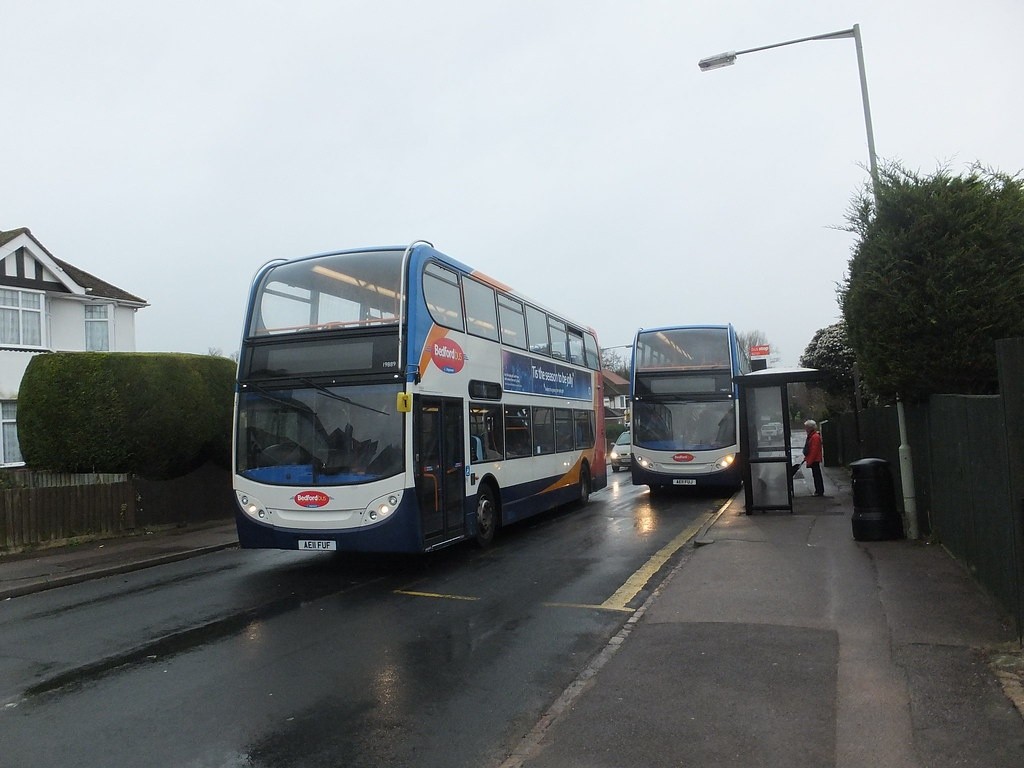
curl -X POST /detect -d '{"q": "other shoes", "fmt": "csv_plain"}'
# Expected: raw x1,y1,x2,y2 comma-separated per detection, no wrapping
811,492,819,496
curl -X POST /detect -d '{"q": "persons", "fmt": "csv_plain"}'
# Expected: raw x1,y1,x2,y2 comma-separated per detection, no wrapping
345,432,392,474
803,420,824,498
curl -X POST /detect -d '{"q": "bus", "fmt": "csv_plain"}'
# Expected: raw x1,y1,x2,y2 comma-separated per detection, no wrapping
229,237,607,555
627,323,756,492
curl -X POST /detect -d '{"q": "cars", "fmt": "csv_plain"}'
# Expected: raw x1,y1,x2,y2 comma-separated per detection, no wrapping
760,421,783,439
609,431,634,473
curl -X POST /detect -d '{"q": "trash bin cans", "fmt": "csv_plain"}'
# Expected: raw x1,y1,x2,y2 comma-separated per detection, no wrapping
849,457,905,542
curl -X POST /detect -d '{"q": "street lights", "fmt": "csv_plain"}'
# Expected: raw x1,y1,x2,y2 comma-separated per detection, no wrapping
697,24,921,541
